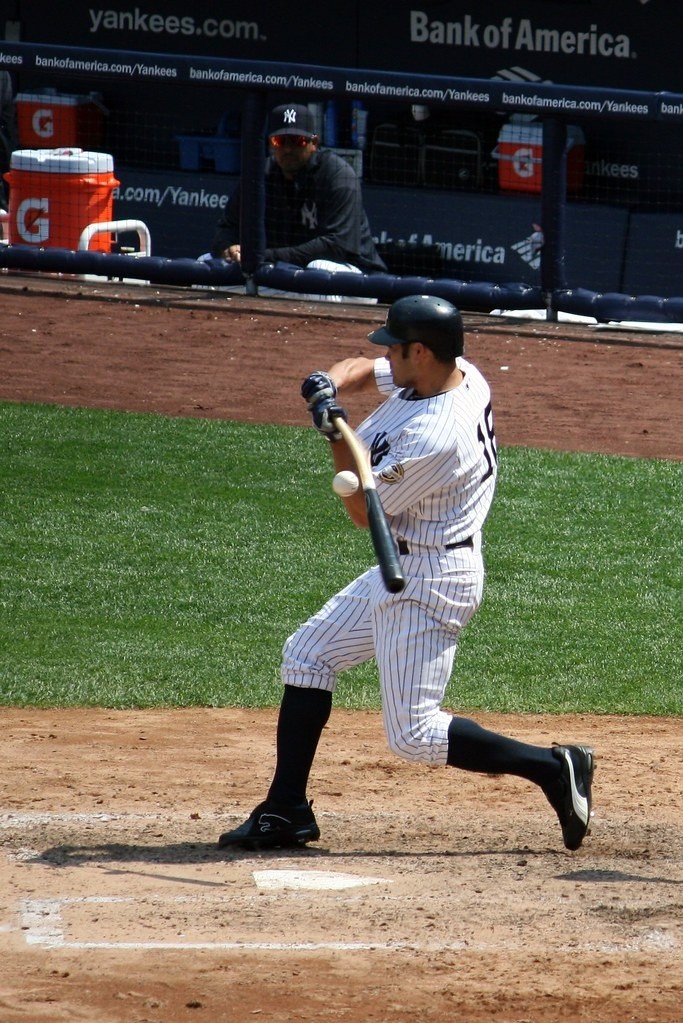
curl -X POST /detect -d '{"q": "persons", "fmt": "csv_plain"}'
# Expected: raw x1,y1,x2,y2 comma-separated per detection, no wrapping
219,293,595,851
224,104,382,308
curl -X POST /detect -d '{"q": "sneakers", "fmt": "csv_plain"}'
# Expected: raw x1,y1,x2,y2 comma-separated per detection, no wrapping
540,740,594,851
219,798,320,849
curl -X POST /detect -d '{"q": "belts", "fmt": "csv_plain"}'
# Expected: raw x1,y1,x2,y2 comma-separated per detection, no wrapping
398,536,473,555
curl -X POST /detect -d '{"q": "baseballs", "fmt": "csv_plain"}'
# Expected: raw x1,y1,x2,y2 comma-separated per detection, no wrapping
332,470,359,497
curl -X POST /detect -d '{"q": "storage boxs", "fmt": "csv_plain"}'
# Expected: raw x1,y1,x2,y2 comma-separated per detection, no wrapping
12,88,110,148
491,122,586,192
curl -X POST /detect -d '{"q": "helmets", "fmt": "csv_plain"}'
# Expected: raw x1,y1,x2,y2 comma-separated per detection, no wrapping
367,295,464,354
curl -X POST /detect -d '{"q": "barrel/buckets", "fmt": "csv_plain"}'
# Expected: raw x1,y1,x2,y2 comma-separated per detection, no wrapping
3,147,120,255
489,122,584,192
14,92,78,151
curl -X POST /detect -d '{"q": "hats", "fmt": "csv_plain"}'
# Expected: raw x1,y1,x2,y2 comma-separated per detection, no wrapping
268,104,315,138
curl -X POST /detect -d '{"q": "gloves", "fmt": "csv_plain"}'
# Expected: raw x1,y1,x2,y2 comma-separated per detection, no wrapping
301,370,338,402
310,402,348,442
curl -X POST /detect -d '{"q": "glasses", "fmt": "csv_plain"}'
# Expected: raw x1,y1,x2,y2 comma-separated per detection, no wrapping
271,134,314,147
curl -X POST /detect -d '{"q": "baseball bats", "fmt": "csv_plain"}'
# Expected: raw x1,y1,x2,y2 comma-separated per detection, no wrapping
307,375,406,594
